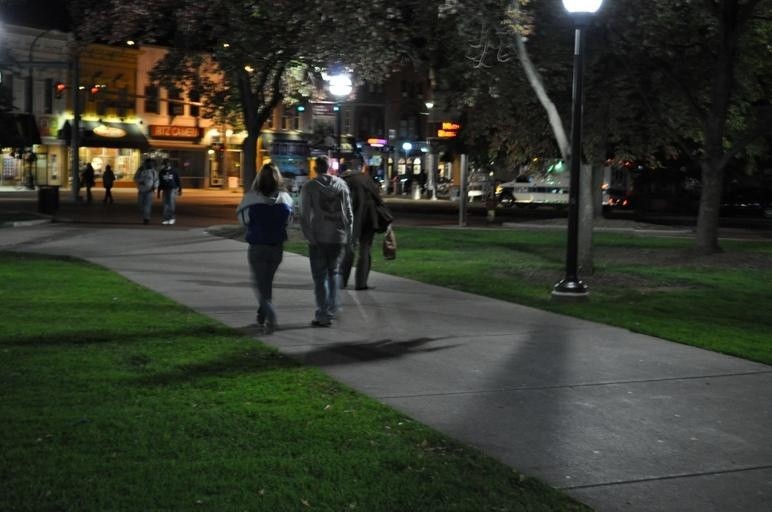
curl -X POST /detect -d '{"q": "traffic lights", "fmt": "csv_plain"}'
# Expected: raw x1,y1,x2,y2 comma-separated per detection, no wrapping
297,104,305,112
88,84,97,103
54,82,63,99
333,105,340,112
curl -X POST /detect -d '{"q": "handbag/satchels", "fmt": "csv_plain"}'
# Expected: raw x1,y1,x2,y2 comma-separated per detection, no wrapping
382,223,397,260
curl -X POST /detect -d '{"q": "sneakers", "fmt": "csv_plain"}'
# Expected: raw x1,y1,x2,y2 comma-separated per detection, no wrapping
161,220,169,224
168,218,175,225
312,318,331,327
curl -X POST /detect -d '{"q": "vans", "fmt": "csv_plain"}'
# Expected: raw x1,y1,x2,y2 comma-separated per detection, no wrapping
494,156,630,209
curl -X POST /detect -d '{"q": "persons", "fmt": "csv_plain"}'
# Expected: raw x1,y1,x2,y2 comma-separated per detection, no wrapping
133,159,160,224
338,157,395,291
102,165,116,204
300,156,354,328
235,162,293,334
80,162,95,202
157,159,183,225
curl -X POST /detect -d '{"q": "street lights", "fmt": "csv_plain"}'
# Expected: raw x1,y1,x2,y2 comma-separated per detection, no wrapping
550,1,603,303
